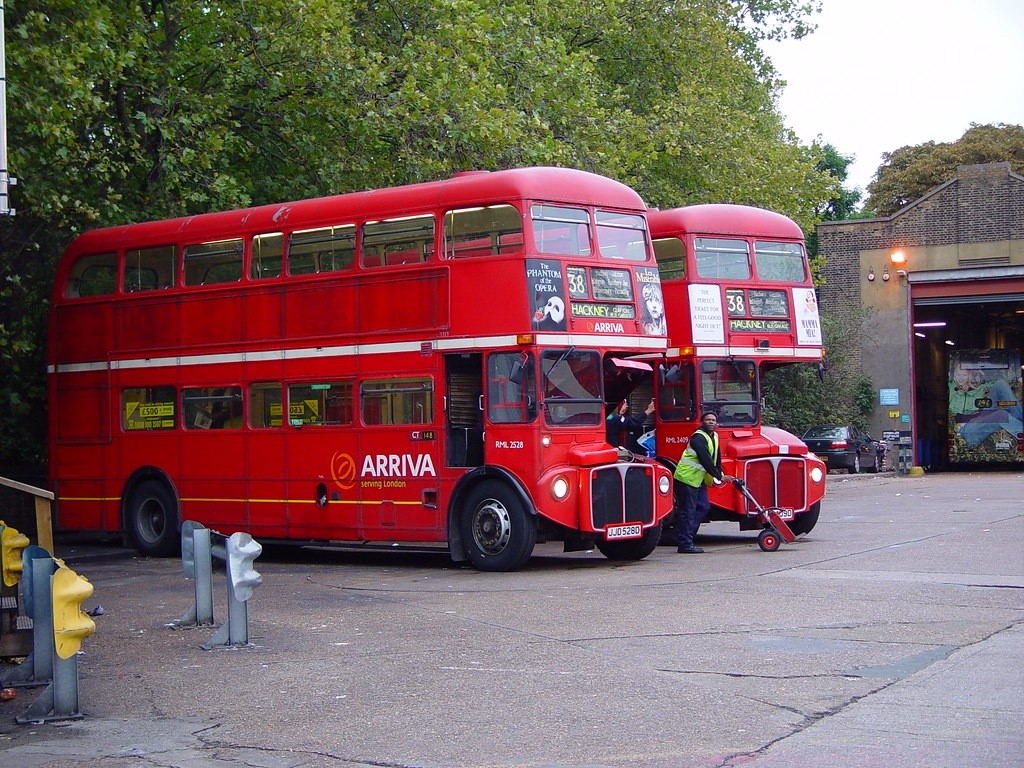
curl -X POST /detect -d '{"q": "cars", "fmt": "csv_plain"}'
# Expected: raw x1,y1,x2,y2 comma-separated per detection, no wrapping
802,424,880,474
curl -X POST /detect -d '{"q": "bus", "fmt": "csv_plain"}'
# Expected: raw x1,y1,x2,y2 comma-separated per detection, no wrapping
948,349,1024,468
327,203,828,536
49,166,684,568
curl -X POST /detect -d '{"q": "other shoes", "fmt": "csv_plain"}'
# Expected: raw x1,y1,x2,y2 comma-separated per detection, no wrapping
677,546,704,554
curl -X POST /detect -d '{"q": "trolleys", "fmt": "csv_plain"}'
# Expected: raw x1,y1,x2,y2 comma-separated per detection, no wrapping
721,473,796,552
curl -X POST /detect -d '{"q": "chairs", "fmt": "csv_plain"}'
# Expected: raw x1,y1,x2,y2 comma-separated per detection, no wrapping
448,375,484,466
495,375,521,422
628,383,655,450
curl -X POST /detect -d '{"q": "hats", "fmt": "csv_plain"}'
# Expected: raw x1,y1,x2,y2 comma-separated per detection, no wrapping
700,412,718,422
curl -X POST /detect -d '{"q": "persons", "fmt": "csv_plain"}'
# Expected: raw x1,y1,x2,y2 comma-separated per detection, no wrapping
673,411,735,553
802,291,819,337
947,361,1024,447
603,399,655,448
